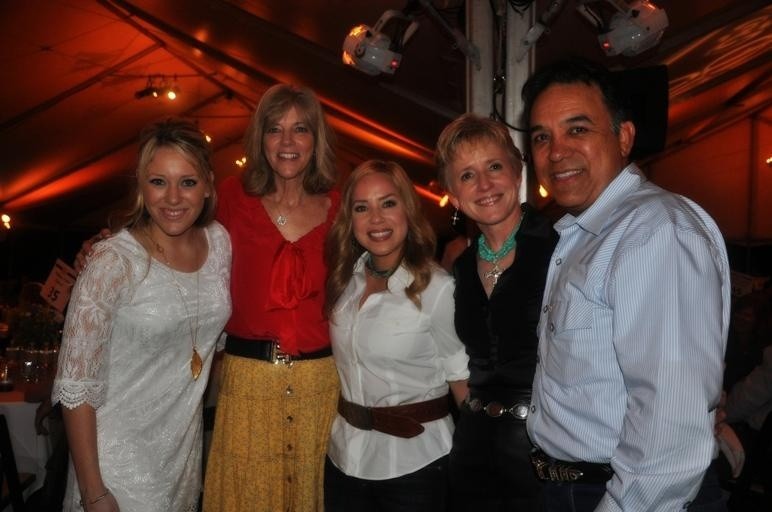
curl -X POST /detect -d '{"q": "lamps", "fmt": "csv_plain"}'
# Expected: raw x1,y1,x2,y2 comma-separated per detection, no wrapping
130,73,181,104
518,0,668,63
343,2,477,81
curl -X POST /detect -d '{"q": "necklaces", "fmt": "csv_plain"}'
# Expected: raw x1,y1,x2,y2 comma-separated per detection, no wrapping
365,257,395,279
153,230,202,382
477,213,524,288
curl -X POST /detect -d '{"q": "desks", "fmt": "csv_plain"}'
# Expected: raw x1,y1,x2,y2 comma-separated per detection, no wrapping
1,389,68,512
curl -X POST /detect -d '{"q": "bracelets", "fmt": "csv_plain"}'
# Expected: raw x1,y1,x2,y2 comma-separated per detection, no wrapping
80,489,108,506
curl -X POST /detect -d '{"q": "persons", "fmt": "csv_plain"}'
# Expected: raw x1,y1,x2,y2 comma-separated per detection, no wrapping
520,56,731,512
73,81,342,512
35,399,68,512
719,292,772,512
272,196,300,226
50,115,232,512
324,159,470,512
433,112,727,512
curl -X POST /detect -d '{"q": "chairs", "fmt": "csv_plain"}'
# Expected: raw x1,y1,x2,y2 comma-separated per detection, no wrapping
1,414,41,511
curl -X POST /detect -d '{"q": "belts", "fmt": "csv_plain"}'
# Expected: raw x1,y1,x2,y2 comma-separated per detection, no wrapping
223,334,333,368
519,435,601,483
335,392,454,439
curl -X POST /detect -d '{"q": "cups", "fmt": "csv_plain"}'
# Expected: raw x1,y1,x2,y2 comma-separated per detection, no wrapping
1,342,61,391
